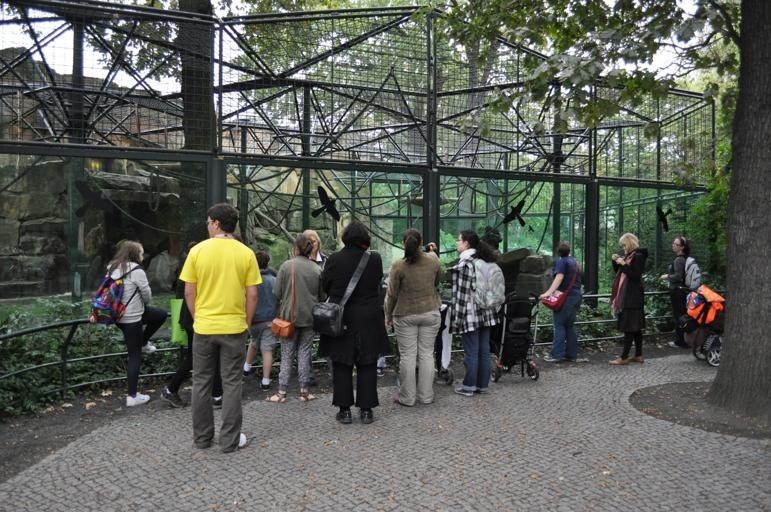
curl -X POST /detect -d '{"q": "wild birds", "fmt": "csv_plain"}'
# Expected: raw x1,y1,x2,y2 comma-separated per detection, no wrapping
657,206,672,232
502,199,526,228
311,186,340,223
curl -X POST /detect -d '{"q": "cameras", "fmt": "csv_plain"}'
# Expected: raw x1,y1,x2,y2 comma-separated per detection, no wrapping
421,245,433,252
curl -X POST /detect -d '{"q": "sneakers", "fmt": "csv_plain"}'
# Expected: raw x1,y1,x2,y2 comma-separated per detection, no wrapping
393,393,434,407
212,397,223,410
125,391,151,407
159,385,185,409
628,355,644,363
236,432,247,447
260,380,271,392
667,341,680,348
336,410,353,424
359,409,375,424
139,341,157,354
543,355,561,362
242,371,251,377
608,356,631,365
455,387,489,397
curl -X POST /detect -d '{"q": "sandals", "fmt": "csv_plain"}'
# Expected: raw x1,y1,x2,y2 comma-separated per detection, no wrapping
299,391,317,402
265,392,287,403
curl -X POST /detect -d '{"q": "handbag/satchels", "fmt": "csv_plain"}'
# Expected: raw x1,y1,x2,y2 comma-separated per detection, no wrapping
271,316,295,338
169,296,190,349
309,300,345,339
542,288,567,312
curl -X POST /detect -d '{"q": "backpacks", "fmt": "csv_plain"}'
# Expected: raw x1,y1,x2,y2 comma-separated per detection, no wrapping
465,254,506,311
90,261,145,326
684,255,701,291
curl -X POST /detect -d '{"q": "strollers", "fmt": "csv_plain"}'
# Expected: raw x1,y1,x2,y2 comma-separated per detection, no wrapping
490,290,543,384
395,298,454,384
683,284,726,370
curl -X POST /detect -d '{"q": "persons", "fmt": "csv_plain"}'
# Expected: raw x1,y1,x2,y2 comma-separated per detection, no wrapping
243,251,278,392
160,235,245,409
318,220,392,425
377,357,387,377
83,225,184,291
265,234,315,403
304,229,328,271
607,233,649,365
106,241,168,407
539,243,582,362
446,230,499,397
483,224,502,254
383,228,440,407
659,235,691,348
177,200,264,454
172,240,199,298
